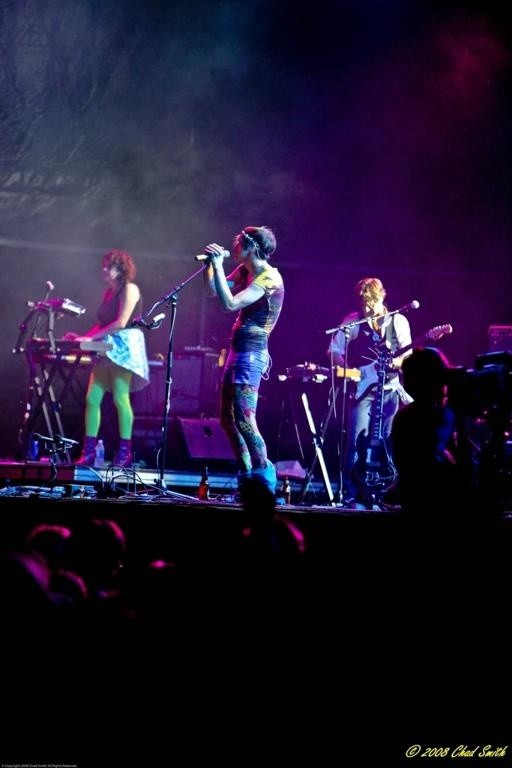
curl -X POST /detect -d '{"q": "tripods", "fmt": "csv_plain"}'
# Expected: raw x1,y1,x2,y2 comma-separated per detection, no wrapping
84,262,209,502
295,309,402,510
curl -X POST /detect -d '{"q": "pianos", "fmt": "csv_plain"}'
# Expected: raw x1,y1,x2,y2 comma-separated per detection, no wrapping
26,338,112,352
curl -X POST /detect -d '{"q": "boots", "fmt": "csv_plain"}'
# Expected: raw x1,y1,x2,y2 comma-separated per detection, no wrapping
75,435,97,464
113,438,133,466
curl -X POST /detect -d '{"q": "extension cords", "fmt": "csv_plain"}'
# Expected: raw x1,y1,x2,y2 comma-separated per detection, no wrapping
97,489,125,498
66,484,94,497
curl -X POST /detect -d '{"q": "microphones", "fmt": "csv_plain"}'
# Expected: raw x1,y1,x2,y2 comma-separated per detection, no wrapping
399,300,420,309
34,433,55,443
195,251,231,261
54,434,78,445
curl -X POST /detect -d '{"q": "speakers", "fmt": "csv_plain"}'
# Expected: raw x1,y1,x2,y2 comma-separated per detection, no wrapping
165,416,246,474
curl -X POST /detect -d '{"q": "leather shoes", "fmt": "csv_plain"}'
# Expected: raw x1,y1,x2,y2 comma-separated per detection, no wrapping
366,493,401,510
341,497,356,506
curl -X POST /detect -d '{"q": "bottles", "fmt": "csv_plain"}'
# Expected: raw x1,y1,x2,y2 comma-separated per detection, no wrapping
280,474,291,504
198,465,209,502
94,440,104,467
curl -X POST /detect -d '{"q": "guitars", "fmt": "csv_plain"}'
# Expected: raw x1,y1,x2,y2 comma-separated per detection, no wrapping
348,323,453,404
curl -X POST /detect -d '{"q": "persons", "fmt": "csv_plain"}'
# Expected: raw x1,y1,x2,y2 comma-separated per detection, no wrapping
327,278,414,510
61,248,151,470
380,347,457,509
204,226,285,495
0,475,304,622
